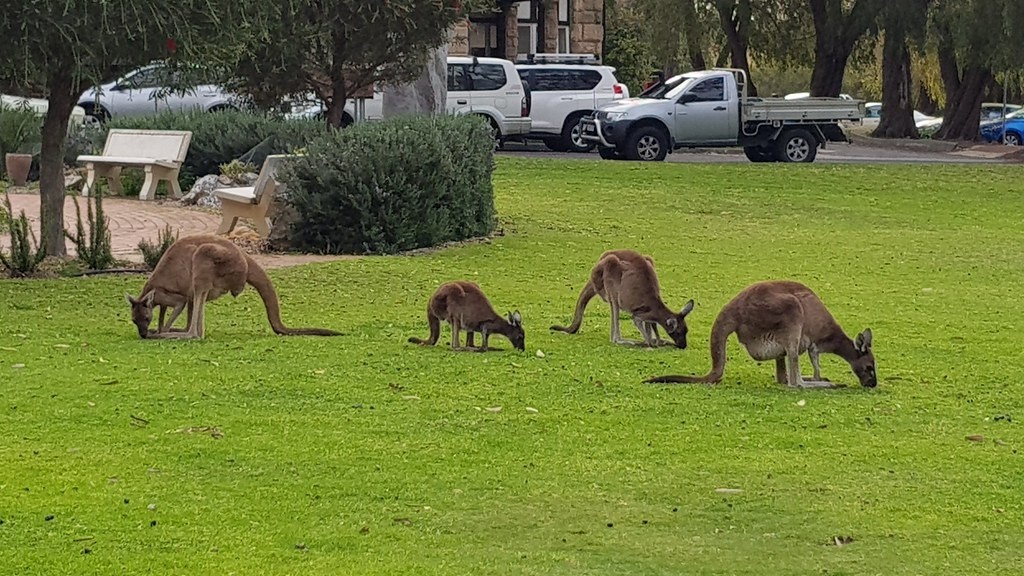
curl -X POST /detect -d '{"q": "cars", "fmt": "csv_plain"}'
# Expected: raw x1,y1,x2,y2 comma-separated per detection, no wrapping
74,60,249,122
843,102,927,138
979,108,1024,148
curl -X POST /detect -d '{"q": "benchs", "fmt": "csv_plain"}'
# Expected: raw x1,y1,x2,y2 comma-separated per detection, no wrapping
211,153,303,237
76,128,192,201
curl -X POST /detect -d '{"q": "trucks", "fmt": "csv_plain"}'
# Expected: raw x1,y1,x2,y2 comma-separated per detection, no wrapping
577,66,866,163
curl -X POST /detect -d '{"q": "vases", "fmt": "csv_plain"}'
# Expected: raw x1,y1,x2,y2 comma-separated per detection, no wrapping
6,152,32,187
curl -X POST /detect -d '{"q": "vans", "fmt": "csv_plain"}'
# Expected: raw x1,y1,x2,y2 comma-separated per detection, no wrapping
283,52,533,149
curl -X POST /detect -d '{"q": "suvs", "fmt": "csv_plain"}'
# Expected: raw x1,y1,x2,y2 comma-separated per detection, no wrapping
502,54,630,153
